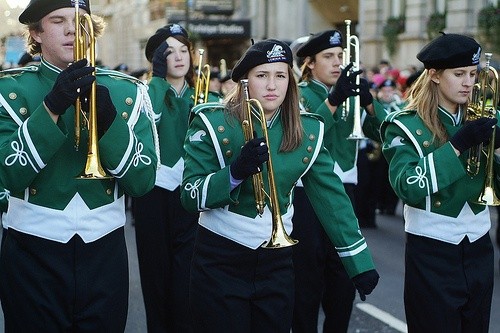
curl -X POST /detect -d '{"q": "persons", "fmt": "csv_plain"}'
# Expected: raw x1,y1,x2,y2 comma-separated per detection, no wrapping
132,23,224,333
0,24,500,227
180,38,380,333
379,31,500,333
0,0,158,333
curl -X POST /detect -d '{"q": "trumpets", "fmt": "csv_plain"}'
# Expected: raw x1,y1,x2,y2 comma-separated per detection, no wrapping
467,52,500,206
341,19,368,139
189,49,210,106
71,0,114,180
240,79,299,248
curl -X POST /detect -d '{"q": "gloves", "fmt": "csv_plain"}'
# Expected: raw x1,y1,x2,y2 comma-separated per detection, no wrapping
230,132,269,181
328,62,373,107
152,40,171,78
450,117,500,153
352,269,379,302
43,58,97,115
73,85,118,140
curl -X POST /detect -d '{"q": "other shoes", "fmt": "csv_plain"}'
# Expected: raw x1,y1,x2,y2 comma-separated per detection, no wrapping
375,207,396,216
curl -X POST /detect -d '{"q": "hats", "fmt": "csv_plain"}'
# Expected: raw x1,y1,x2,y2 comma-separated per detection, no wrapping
415,31,482,70
195,65,232,82
94,60,149,78
18,52,42,65
19,0,90,25
296,29,344,58
231,38,293,83
145,24,189,61
368,61,425,90
